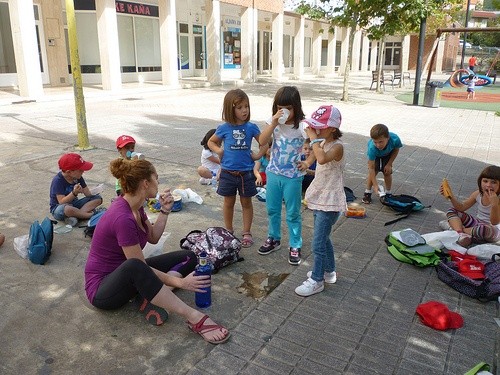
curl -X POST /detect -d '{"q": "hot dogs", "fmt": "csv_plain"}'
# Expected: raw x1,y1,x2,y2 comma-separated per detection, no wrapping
441,177,453,199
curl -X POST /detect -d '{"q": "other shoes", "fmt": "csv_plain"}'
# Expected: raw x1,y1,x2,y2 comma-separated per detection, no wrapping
458,233,473,246
94,207,107,212
64,216,78,226
439,220,453,231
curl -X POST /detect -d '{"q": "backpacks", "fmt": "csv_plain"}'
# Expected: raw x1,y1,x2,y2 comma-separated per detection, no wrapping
380,192,423,214
436,253,500,298
26,216,58,265
387,226,451,267
179,226,241,270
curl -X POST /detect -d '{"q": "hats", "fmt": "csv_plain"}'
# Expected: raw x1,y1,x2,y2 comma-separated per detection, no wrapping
58,153,93,171
457,258,486,279
448,249,476,261
417,301,464,330
301,105,341,129
116,135,135,148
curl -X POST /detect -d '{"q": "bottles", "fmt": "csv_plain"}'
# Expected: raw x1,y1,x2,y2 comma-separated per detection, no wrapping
211,171,218,190
194,250,211,308
126,151,145,161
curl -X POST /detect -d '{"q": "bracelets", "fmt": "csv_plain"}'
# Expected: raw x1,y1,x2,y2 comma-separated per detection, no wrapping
160,209,171,215
72,190,78,196
309,139,325,148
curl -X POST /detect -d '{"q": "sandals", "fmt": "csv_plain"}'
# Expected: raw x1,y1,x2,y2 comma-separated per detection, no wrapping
241,232,253,247
187,315,232,344
134,295,169,325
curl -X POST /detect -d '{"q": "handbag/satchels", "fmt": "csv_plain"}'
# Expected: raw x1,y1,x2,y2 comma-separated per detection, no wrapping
84,211,106,236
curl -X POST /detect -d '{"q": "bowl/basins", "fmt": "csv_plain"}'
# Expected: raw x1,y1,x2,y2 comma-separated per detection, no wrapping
346,204,368,218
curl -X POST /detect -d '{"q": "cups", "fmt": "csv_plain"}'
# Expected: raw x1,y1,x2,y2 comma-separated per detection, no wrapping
278,108,291,124
302,126,319,139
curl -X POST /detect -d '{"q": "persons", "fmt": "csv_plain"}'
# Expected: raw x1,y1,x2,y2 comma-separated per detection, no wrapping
469,55,477,71
362,124,403,204
84,157,232,345
252,137,274,187
49,151,103,227
256,86,317,265
197,129,224,186
464,74,478,99
300,137,318,200
207,88,270,249
440,166,500,248
114,135,136,197
294,104,349,298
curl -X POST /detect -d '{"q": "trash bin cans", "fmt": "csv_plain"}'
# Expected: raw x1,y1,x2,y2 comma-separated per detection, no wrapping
422,80,444,108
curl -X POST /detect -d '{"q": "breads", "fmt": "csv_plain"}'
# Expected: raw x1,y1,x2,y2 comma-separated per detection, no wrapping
347,208,364,216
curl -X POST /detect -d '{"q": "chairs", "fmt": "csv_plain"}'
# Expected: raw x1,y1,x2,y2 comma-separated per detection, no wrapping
393,68,411,88
369,70,394,91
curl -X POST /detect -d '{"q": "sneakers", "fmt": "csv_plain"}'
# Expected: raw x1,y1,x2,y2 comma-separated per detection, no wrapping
288,247,301,264
362,192,372,202
294,278,324,296
257,237,280,254
307,270,336,283
